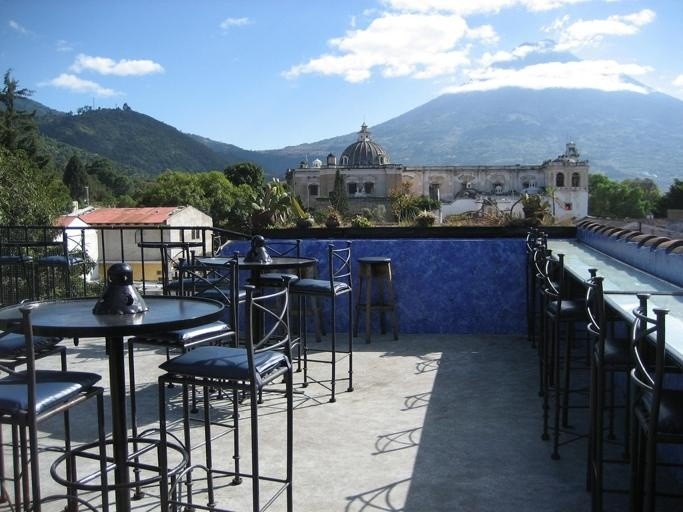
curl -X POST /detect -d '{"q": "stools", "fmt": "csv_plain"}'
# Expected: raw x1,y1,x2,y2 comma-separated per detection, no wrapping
525,226,683,512
0,231,354,512
352,256,399,344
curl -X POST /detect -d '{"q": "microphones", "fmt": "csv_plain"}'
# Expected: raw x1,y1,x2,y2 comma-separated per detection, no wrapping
352,256,399,344
